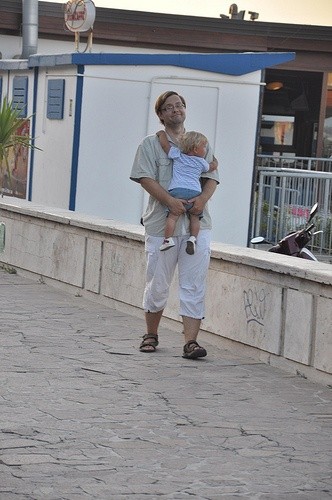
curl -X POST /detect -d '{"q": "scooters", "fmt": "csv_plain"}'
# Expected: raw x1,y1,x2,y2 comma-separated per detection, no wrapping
249,200,321,261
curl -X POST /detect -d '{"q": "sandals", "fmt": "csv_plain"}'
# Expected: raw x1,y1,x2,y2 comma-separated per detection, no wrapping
182,340,207,359
140,333,158,353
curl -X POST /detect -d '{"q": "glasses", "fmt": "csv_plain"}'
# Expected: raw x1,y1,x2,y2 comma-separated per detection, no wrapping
161,103,185,112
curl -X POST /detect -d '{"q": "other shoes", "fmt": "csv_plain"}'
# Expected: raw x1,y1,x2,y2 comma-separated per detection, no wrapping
160,237,175,251
186,236,197,255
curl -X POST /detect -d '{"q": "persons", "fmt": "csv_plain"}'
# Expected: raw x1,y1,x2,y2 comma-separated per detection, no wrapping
130,91,219,358
157,131,218,255
